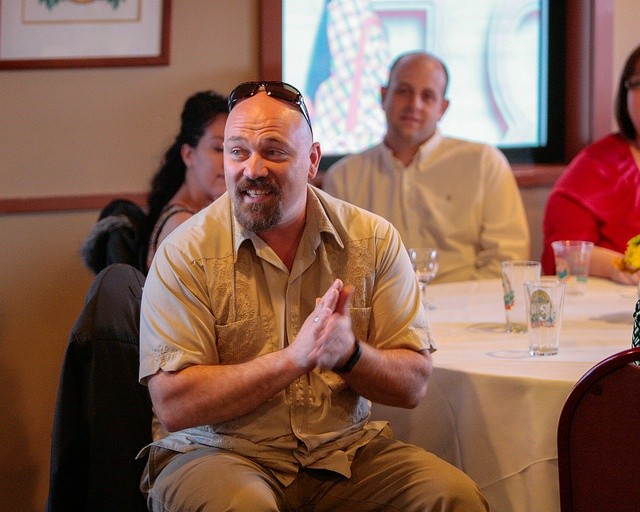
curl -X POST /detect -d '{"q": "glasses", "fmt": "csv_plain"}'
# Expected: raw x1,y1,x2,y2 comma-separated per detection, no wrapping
229,81,312,127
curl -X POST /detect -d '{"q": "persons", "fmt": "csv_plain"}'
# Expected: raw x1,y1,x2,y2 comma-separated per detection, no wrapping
323,50,532,284
142,89,230,272
138,80,491,512
542,43,640,285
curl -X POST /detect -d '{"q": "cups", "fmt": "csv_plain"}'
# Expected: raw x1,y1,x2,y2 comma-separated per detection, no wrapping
552,240,594,295
523,279,566,356
500,260,541,333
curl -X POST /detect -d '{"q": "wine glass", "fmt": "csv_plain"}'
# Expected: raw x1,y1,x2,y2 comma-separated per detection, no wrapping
408,247,439,313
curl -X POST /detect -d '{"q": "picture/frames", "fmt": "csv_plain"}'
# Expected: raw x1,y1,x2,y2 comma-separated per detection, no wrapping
0,0,172,70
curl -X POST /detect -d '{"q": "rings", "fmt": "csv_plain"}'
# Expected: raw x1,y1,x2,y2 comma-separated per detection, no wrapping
313,316,320,323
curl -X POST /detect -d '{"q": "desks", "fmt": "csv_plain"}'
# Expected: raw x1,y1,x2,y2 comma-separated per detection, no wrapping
370,275,640,512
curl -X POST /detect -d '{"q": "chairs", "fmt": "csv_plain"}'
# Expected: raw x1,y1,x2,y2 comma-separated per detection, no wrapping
556,346,640,512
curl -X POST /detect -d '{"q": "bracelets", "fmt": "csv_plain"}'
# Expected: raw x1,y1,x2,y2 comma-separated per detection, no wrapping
328,339,362,374
